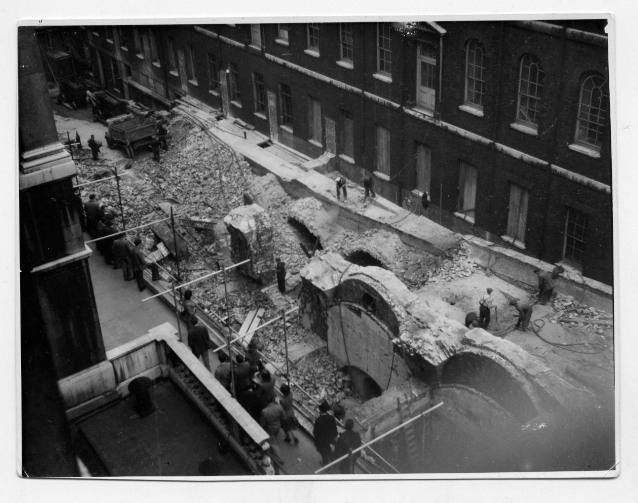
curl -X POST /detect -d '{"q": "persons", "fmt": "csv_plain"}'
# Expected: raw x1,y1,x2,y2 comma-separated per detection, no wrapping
215,352,231,392
363,174,376,197
508,297,533,331
275,257,286,293
187,315,213,373
334,175,347,202
150,135,160,161
156,125,169,151
232,340,300,464
335,417,361,473
180,289,196,329
86,134,100,159
84,194,147,291
532,269,553,305
311,401,337,466
477,286,494,329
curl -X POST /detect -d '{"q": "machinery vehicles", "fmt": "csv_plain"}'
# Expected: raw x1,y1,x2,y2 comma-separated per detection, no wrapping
105,115,168,158
